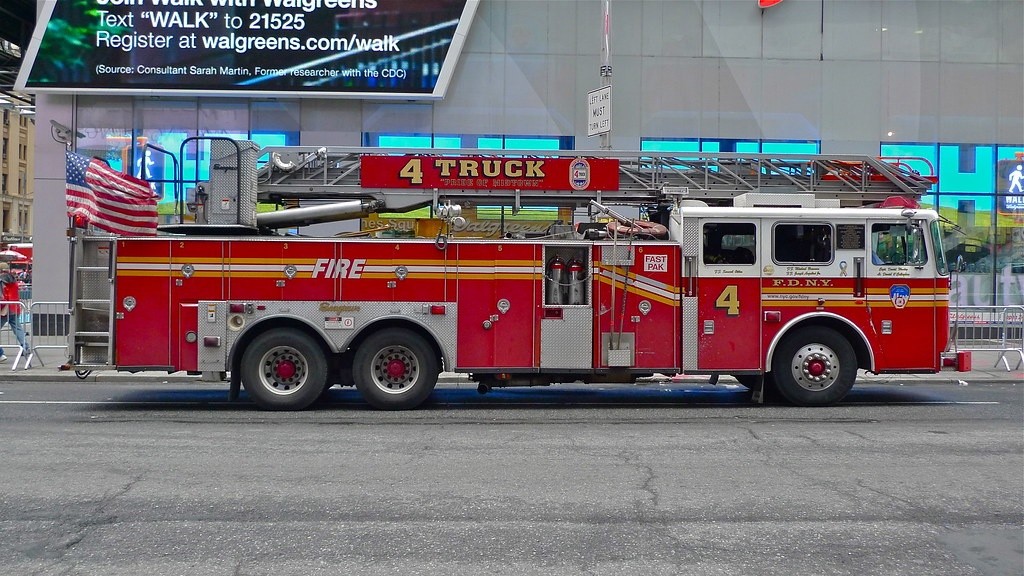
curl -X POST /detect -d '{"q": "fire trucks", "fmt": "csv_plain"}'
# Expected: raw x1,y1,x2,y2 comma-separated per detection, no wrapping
59,130,973,409
5,242,33,296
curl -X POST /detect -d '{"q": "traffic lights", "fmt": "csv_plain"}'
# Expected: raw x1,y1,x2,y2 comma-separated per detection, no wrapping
124,144,164,200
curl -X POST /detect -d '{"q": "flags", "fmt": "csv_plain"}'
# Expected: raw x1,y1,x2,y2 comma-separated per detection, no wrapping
65,149,158,238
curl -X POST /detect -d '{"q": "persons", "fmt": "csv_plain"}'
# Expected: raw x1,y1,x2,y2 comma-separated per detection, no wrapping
12,264,32,284
0,262,31,363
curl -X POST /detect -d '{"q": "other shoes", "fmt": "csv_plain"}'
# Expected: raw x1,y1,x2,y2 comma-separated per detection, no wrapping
21,348,31,356
0,355,8,362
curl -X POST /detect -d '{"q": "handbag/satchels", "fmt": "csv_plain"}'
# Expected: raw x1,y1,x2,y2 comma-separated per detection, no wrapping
0,300,8,316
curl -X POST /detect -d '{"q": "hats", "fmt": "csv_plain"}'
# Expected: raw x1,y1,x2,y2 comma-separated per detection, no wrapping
0,263,10,272
